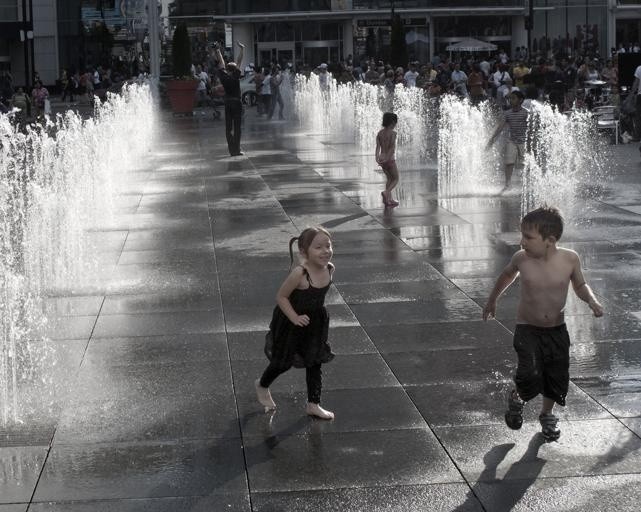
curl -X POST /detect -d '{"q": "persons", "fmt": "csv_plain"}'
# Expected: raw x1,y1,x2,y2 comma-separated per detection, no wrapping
211,40,245,157
2,45,640,166
254,228,335,420
483,90,532,197
481,206,604,440
375,111,401,207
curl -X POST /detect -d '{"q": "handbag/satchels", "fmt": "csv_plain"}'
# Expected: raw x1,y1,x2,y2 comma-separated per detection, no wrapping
499,80,506,85
620,93,638,114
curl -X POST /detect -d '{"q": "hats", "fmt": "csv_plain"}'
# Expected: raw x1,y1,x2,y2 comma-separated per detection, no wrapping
432,79,451,90
317,63,328,69
244,63,257,72
594,58,599,61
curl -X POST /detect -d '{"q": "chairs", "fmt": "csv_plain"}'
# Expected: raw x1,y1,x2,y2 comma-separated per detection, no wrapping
592,105,622,145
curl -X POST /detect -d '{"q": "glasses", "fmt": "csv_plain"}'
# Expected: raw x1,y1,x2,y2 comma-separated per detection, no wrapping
588,64,593,67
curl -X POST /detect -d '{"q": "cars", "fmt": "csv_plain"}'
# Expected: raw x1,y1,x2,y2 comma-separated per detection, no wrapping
239,74,257,105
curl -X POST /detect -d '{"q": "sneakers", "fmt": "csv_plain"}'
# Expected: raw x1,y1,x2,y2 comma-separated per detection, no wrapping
505,389,525,431
538,411,562,441
